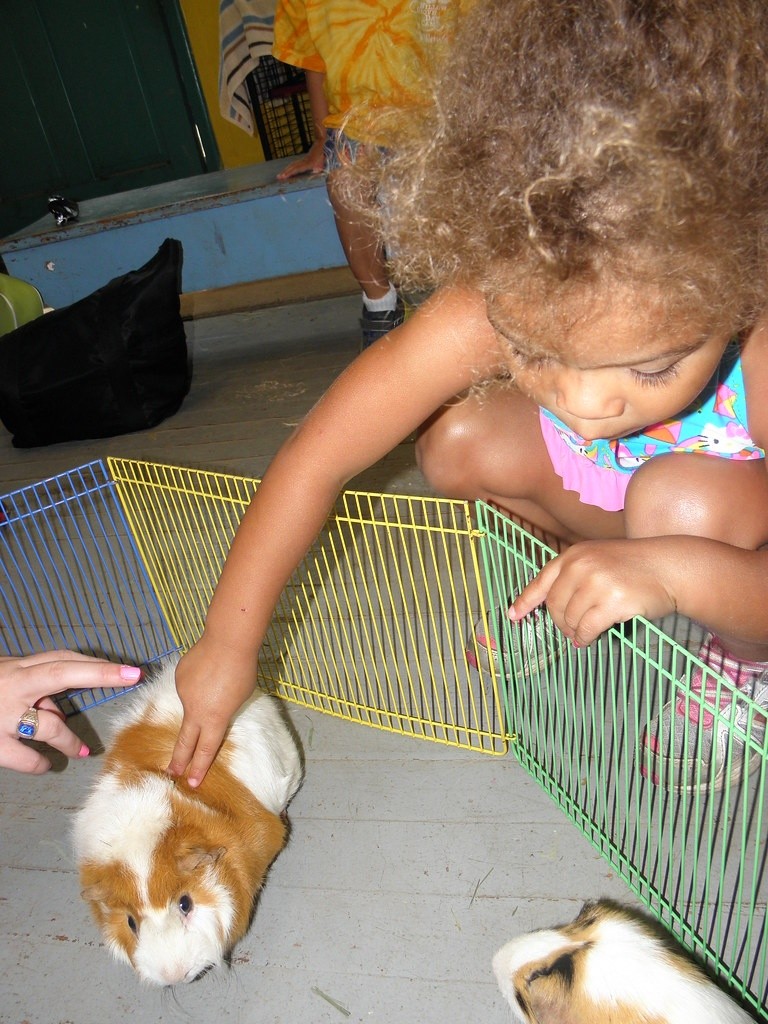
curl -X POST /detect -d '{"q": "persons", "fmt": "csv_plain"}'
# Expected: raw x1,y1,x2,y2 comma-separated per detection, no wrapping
268,0,486,349
0,649,140,776
172,0,768,791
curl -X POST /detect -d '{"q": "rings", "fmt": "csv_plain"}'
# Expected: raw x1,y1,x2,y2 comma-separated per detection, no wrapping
16,708,39,739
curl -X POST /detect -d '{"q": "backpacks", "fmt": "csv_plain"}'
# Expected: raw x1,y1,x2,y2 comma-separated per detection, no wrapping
1,238,195,453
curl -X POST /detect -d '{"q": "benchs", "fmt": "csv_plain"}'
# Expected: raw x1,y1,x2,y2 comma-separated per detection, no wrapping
0,145,394,320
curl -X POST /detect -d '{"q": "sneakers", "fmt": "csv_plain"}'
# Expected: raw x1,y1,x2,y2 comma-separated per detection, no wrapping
362,301,407,354
464,591,571,681
634,636,768,790
